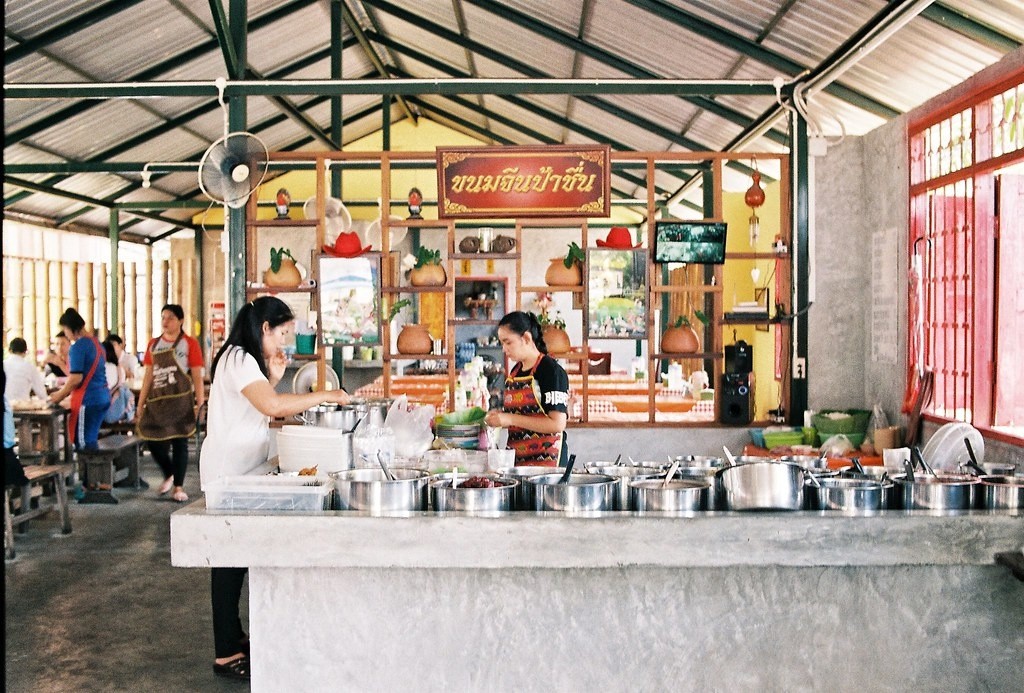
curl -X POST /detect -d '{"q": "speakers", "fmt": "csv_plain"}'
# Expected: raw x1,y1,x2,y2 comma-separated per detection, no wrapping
724,340,752,372
719,371,756,426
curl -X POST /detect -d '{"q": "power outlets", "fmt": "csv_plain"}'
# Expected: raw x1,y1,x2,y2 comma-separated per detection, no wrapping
221,231,230,253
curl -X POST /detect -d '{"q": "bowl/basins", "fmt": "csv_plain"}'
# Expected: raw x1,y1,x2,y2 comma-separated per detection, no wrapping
791,445,811,455
818,432,864,448
812,412,869,434
763,432,803,449
861,427,899,454
277,429,349,475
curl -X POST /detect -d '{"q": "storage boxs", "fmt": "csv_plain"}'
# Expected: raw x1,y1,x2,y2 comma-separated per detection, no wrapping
201,475,335,511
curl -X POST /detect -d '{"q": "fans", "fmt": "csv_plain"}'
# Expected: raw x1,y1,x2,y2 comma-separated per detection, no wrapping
365,215,409,251
198,132,268,209
291,361,340,394
303,198,352,245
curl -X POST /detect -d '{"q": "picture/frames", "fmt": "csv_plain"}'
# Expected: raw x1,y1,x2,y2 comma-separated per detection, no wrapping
436,144,610,219
754,288,769,332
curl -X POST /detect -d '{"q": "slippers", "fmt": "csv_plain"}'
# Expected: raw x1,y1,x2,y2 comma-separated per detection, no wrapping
170,491,189,501
159,475,174,493
213,655,251,680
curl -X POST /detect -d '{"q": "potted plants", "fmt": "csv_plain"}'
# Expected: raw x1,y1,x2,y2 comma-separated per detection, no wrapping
660,310,710,352
545,241,586,286
389,299,432,354
537,310,570,354
405,246,447,287
265,247,302,287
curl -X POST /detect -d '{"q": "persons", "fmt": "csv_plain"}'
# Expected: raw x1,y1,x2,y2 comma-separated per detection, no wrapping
485,311,568,467
0,304,206,517
200,296,354,683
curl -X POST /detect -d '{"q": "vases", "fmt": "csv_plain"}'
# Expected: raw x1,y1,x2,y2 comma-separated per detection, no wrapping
295,333,383,361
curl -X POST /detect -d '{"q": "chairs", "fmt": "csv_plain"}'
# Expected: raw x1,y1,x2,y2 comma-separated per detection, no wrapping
578,352,612,376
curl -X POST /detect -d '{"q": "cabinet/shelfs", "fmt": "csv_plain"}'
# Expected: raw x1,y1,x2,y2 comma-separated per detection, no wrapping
245,152,790,427
448,277,509,383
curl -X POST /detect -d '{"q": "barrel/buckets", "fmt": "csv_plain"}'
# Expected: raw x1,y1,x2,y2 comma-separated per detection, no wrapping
295,399,393,430
332,450,1024,512
296,335,316,354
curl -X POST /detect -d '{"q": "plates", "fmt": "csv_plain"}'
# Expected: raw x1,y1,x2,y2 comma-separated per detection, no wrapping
433,406,486,449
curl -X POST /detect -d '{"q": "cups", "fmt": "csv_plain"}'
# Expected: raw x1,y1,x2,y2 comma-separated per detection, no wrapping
361,348,372,360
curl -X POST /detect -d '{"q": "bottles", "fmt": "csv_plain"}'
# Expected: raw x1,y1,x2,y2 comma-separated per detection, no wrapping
668,361,682,389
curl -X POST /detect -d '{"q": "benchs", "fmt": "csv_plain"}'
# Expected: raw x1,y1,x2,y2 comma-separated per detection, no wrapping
72,434,151,505
2,466,72,560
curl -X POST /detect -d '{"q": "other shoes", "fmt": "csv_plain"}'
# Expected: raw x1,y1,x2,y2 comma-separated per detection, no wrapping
74,487,87,501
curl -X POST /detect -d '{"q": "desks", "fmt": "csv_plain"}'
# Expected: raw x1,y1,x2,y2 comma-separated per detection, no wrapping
353,370,715,422
12,407,72,498
